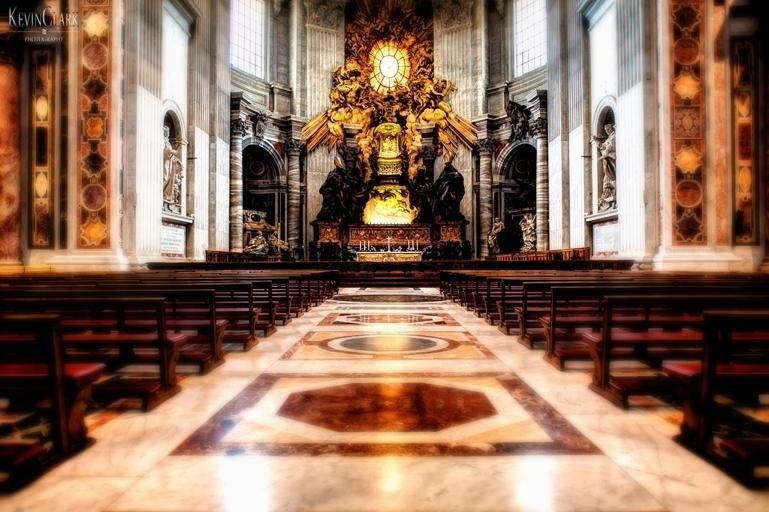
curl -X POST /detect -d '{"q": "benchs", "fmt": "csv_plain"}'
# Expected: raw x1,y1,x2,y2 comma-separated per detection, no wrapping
0,268,339,496
438,268,769,493
